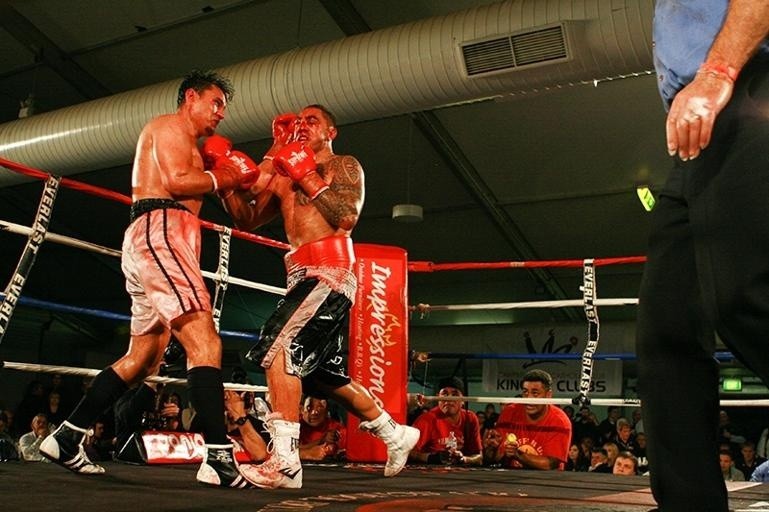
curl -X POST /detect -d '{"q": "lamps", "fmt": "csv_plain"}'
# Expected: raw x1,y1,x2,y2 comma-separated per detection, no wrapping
391,118,424,223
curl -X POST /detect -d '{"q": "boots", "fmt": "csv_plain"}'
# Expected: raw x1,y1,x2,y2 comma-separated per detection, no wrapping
359,410,420,477
239,412,303,489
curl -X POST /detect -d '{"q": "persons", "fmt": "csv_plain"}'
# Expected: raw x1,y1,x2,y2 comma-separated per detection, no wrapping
1,390,198,462
202,105,421,490
483,371,576,468
635,1,768,512
38,71,298,491
297,396,347,464
717,410,769,483
410,376,485,467
474,393,522,466
114,370,179,464
554,404,650,477
223,390,267,464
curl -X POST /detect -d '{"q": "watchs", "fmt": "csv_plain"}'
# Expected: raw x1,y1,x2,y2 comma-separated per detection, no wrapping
232,417,250,426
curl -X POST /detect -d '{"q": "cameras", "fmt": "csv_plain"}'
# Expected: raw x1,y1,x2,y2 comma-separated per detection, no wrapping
228,367,255,409
165,337,184,367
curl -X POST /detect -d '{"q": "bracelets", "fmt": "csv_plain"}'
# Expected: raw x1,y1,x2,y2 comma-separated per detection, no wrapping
694,63,739,84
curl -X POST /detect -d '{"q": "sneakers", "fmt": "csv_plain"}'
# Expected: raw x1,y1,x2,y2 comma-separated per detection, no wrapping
38,420,106,474
196,444,256,489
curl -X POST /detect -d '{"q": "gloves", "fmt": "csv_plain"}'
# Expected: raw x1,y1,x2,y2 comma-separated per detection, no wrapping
204,113,330,201
